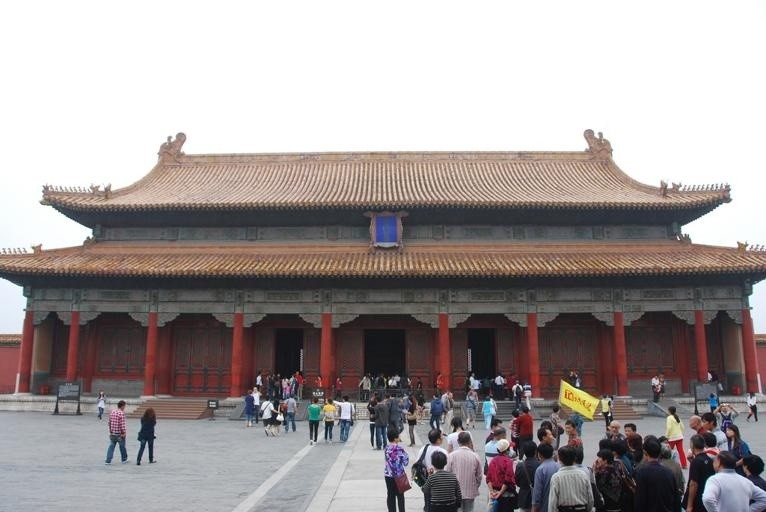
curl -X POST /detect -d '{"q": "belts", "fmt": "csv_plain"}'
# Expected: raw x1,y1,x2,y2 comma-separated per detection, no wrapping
558,506,588,511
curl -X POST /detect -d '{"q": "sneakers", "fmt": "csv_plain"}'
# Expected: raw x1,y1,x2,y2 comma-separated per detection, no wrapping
310,439,317,446
338,439,347,443
122,457,129,464
369,446,385,450
324,440,332,445
149,460,157,463
407,443,415,448
264,429,281,437
136,463,141,465
105,462,112,465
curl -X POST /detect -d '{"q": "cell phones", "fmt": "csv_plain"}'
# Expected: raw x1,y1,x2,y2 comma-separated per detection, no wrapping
722,403,728,407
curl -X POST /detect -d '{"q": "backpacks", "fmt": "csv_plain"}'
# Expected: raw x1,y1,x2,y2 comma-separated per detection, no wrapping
719,412,733,434
516,383,522,396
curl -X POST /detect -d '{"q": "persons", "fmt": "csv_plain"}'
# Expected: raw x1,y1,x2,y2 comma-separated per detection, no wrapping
591,392,765,512
96,390,106,419
105,400,129,464
383,404,606,512
358,372,531,450
246,369,356,444
136,408,156,465
651,374,664,402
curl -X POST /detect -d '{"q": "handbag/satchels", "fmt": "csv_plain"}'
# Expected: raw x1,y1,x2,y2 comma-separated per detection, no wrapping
110,434,122,442
351,417,354,426
281,399,289,412
411,444,430,487
394,469,412,496
530,484,534,497
276,413,285,422
258,409,264,418
490,408,497,416
514,493,520,509
619,461,637,497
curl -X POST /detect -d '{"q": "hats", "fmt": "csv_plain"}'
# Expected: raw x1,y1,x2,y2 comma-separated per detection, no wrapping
496,438,516,454
494,428,508,436
